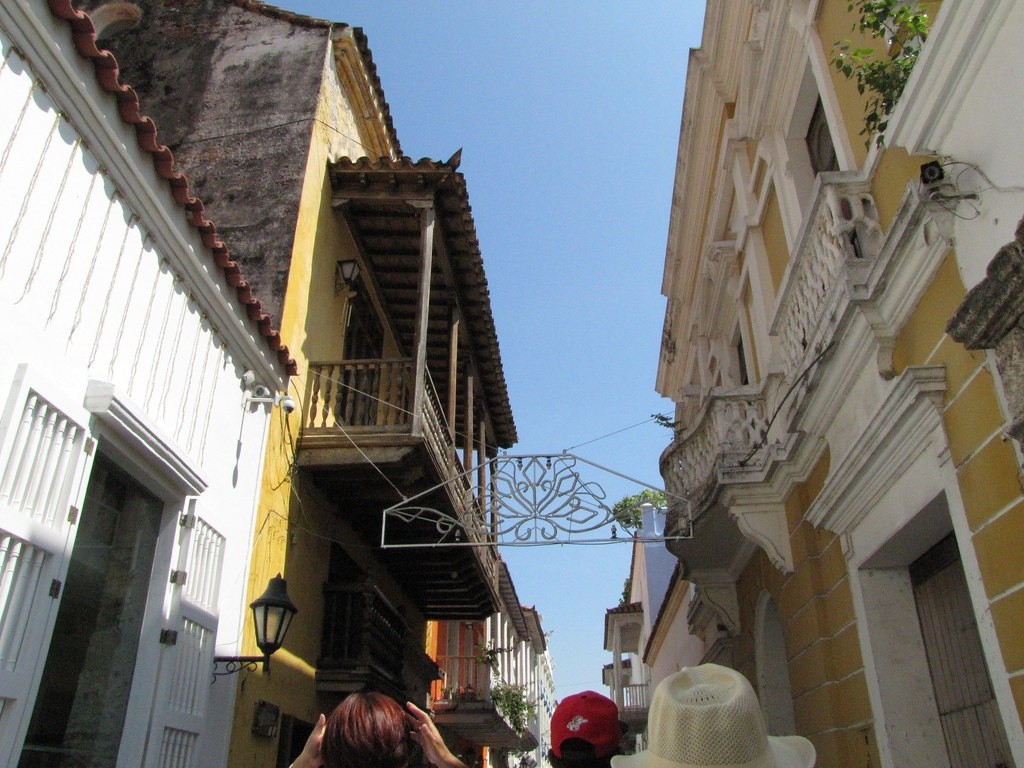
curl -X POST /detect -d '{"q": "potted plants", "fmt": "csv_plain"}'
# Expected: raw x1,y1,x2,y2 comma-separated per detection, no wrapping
449,682,483,701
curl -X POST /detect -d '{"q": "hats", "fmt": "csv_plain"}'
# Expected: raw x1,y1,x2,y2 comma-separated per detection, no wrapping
610,663,817,768
551,690,628,761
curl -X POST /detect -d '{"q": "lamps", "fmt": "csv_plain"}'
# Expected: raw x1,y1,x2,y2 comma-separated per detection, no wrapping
248,574,300,673
337,257,363,286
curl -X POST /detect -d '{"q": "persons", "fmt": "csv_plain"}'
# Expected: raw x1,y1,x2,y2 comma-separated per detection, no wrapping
549,689,625,768
286,690,472,768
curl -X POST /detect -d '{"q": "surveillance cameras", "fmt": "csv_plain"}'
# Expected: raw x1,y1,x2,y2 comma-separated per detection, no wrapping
283,401,295,414
920,161,946,185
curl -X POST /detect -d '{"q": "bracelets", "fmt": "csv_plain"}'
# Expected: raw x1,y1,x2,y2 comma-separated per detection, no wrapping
288,763,296,768
